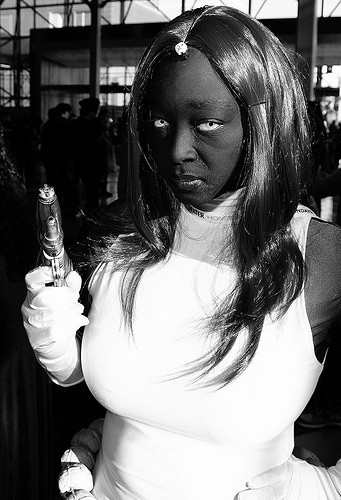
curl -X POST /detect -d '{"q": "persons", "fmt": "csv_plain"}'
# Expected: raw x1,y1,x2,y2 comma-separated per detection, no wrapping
0,96,341,429
22,6,341,499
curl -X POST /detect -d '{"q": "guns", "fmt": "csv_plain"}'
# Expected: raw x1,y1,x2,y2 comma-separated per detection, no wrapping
34,181,74,289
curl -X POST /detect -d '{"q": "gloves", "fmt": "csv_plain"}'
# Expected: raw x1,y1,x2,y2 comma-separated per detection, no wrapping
21,265,90,387
236,452,341,500
